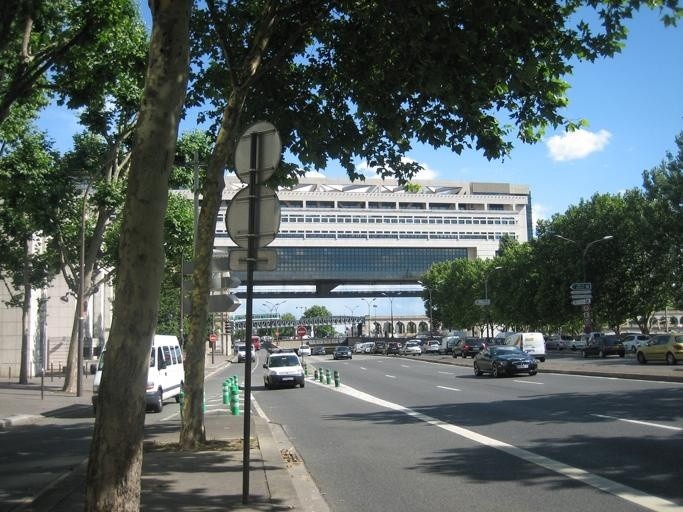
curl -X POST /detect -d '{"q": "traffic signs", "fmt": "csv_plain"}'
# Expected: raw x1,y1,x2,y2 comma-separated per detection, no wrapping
571,299,591,306
569,294,591,299
569,282,591,290
473,298,490,306
570,290,591,294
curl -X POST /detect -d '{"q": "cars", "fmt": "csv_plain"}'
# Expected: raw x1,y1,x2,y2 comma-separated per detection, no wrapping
473,345,537,378
635,333,682,365
579,336,624,359
620,334,651,353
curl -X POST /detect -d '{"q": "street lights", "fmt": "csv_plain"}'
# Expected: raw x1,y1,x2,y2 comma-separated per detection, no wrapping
360,298,376,339
463,265,503,338
258,300,287,347
344,304,358,336
295,305,308,326
381,292,402,338
554,233,615,335
60,148,187,398
416,279,445,334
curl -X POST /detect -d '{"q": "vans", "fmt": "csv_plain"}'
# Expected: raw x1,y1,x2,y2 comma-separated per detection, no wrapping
262,352,305,388
91,333,184,414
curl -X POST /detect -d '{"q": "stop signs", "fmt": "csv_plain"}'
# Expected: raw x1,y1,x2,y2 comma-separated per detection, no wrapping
210,334,216,342
296,327,306,336
